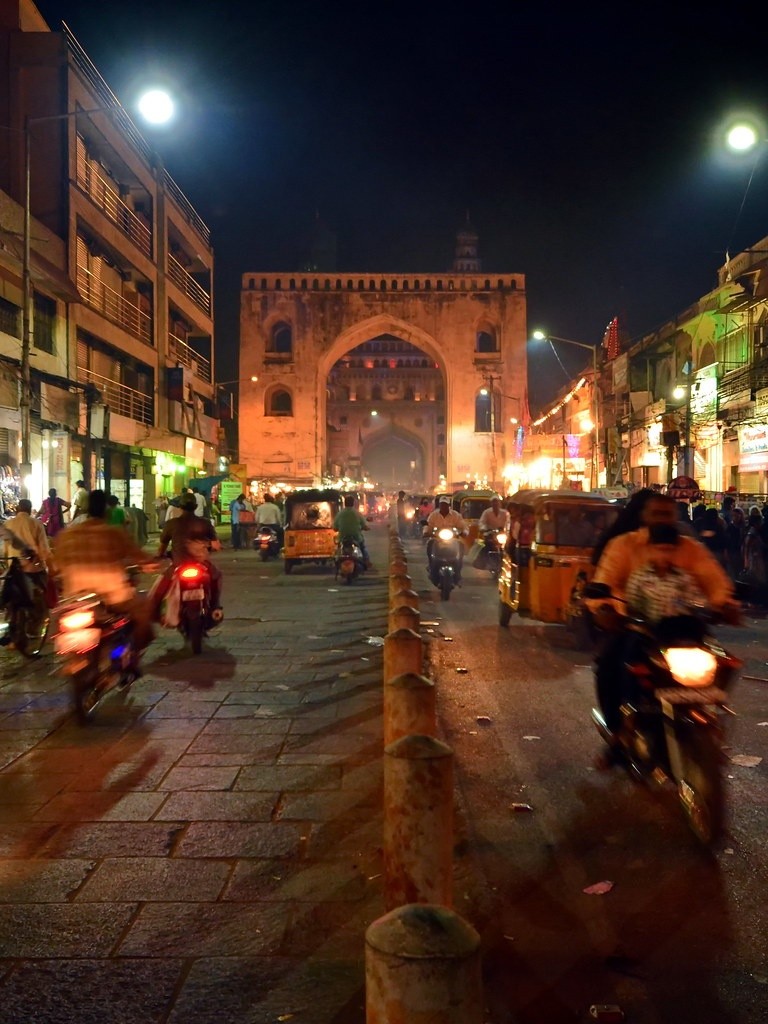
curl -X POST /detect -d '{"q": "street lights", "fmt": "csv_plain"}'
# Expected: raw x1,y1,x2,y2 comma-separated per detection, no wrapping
532,330,600,489
18,86,176,494
212,376,259,417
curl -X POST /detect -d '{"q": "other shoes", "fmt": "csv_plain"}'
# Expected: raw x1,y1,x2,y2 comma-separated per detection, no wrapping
748,602,760,610
8,624,17,632
121,664,141,687
598,733,635,772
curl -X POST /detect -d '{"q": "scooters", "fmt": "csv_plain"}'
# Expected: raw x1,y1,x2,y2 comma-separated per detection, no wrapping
0,554,46,660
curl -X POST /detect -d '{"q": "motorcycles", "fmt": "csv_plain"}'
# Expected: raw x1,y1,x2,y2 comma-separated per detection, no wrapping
153,546,224,657
577,581,746,862
413,489,499,570
279,487,387,575
50,563,148,719
496,487,622,650
336,534,368,585
254,526,279,562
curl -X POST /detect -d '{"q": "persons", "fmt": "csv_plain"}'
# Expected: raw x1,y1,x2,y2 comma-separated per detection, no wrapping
229,493,286,550
3,499,58,609
679,495,768,619
105,494,130,528
0,522,41,563
158,493,227,620
393,487,513,570
585,487,744,775
165,487,223,525
332,494,372,571
51,490,160,668
72,481,90,522
35,488,72,537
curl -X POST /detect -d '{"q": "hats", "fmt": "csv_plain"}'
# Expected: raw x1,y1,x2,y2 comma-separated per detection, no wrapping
490,494,503,501
439,495,451,505
180,493,197,508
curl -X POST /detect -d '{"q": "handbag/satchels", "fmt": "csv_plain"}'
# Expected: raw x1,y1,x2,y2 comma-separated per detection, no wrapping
739,569,754,582
44,573,58,608
144,565,180,628
